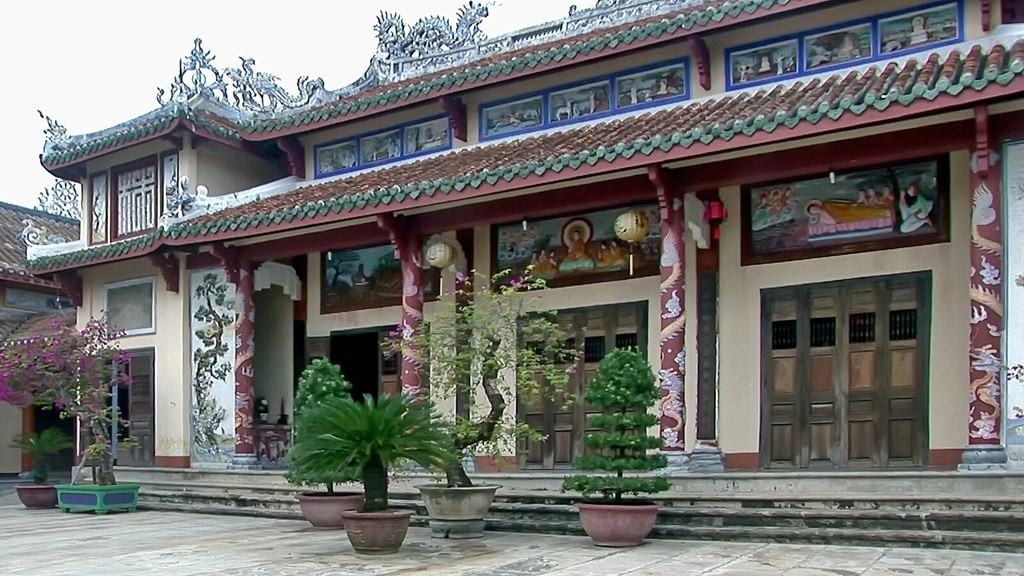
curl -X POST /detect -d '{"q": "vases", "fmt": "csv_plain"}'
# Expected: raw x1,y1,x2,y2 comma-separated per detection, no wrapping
409,481,499,541
57,481,141,513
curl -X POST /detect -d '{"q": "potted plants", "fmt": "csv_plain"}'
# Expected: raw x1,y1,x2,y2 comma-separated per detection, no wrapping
286,388,458,554
295,356,366,528
8,427,70,507
564,347,672,548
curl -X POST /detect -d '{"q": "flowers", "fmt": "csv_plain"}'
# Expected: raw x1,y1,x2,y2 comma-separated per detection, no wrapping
369,266,570,483
0,317,149,485
